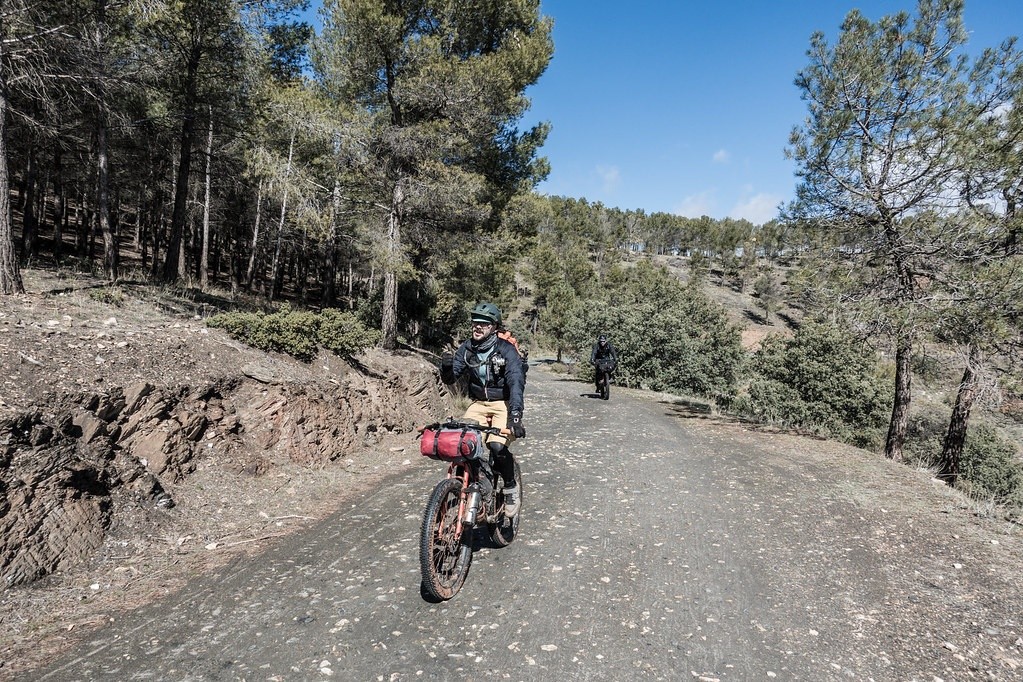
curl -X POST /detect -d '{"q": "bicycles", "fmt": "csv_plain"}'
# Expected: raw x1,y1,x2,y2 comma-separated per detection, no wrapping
593,360,614,399
416,416,523,601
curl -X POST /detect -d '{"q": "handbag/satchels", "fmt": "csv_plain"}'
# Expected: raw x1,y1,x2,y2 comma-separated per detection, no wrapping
420,426,484,461
599,363,615,372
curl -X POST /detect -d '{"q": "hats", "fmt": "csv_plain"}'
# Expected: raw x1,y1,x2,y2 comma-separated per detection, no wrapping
472,316,491,324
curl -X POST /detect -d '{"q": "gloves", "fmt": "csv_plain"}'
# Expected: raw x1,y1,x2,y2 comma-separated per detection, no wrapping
442,343,453,371
614,360,618,364
506,410,526,438
591,360,594,365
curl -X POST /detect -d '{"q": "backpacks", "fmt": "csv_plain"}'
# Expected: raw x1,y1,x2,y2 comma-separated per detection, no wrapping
492,329,529,384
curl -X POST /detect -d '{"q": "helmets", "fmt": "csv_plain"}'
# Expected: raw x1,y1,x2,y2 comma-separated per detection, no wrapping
469,303,502,325
599,335,606,341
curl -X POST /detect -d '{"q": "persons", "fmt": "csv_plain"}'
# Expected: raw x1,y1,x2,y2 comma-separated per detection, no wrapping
591,335,617,393
439,303,527,518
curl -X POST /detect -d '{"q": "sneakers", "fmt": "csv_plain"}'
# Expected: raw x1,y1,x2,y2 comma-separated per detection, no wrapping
502,481,520,517
